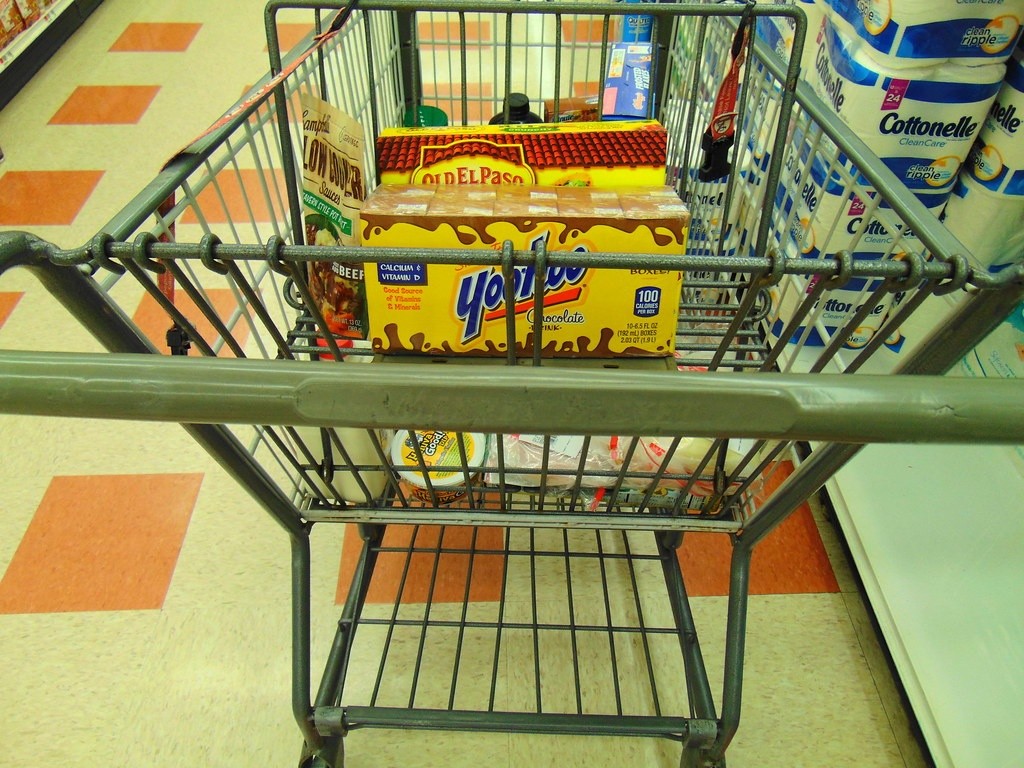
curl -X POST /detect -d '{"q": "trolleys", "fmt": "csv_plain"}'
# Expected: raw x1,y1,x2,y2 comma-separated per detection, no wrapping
1,0,1022,768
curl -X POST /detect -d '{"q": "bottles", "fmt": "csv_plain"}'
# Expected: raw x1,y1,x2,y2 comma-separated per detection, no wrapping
489,93,544,124
290,329,395,501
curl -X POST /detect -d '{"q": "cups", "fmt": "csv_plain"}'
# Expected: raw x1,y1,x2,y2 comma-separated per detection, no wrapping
403,106,448,127
